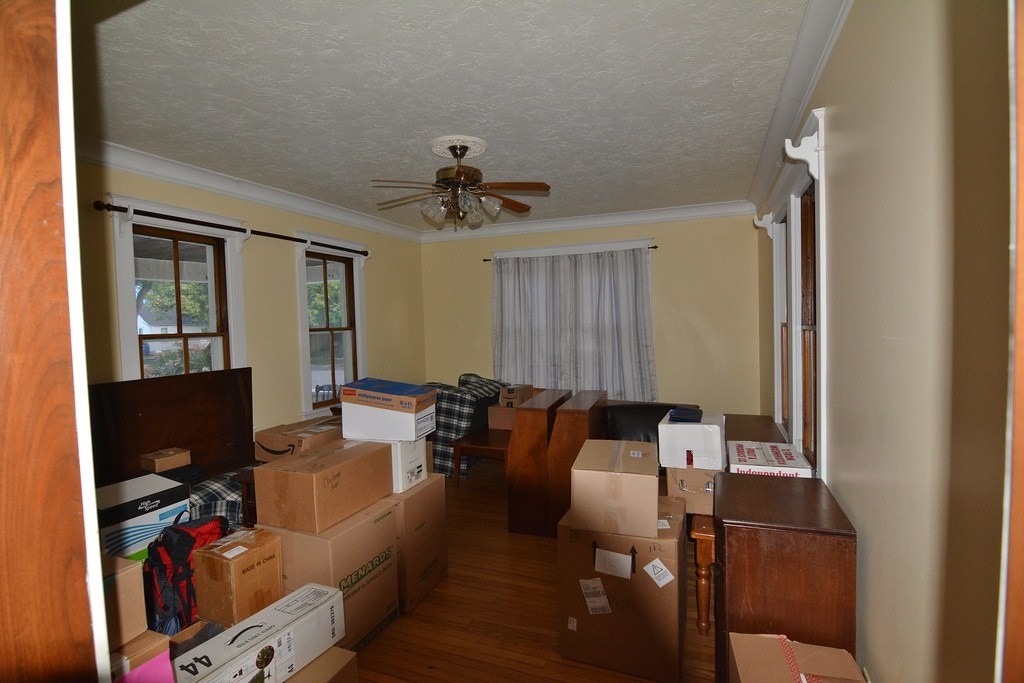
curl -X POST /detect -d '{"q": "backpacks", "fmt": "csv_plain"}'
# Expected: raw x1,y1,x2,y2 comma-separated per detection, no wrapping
143,514,232,636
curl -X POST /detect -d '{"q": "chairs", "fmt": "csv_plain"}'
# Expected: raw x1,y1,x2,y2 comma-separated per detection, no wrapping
315,385,341,401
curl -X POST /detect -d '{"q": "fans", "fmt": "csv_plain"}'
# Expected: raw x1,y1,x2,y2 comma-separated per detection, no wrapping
371,136,550,212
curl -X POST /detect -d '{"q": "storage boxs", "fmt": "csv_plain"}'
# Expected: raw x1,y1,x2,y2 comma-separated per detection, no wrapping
95,372,870,683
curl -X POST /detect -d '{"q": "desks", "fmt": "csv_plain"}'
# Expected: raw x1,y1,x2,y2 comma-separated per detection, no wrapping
725,414,786,443
690,514,715,636
451,427,513,485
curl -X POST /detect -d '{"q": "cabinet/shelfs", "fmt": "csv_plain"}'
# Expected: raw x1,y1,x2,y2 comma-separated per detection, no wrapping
713,472,857,683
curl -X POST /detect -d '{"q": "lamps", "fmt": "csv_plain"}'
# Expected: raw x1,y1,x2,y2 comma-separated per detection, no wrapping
422,183,503,223
